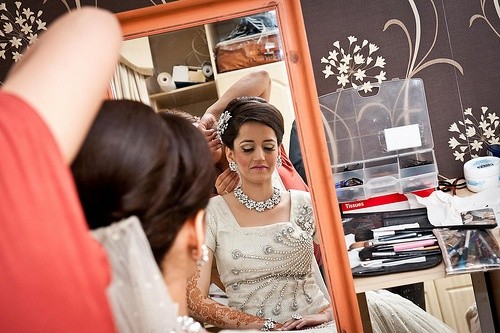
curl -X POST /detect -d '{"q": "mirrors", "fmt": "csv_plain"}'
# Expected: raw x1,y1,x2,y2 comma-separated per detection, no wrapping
78,0,363,333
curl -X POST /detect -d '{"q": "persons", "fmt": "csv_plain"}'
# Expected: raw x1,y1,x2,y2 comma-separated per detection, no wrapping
68,97,208,333
186,96,339,333
0,7,124,333
199,70,323,293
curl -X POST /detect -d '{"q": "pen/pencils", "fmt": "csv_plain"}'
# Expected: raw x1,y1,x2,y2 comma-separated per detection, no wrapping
441,231,500,272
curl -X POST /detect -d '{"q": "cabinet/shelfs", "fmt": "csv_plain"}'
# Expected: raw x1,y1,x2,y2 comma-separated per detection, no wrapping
108,7,308,212
338,178,500,333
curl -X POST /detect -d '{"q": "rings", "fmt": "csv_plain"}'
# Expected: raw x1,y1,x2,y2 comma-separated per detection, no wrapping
292,314,302,320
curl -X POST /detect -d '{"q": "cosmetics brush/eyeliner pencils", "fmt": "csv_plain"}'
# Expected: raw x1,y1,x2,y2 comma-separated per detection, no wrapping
445,229,500,267
347,227,441,269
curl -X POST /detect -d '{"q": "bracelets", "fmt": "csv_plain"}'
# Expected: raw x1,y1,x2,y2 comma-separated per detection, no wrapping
262,318,276,331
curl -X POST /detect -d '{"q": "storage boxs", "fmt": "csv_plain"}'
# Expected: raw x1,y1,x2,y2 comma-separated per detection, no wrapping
318,78,439,204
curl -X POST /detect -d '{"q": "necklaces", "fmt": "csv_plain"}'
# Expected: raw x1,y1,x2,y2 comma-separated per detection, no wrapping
234,185,282,212
